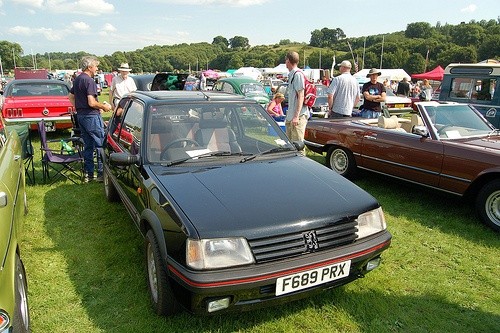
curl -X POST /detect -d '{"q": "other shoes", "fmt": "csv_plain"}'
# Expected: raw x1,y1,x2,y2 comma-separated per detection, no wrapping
87,176,93,183
97,175,103,182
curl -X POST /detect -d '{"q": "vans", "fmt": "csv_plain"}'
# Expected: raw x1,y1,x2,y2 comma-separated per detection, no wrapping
439,63,500,131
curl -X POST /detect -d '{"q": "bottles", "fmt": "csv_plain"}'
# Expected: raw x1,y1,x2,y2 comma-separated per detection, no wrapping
61,139,75,155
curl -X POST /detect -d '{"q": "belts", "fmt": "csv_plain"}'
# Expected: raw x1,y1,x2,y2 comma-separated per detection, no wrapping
343,114,350,117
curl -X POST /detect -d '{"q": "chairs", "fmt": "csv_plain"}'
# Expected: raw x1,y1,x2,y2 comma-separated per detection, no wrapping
7,122,36,187
15,90,28,96
195,111,242,155
68,112,103,163
379,115,407,134
412,114,433,135
34,118,87,186
48,89,61,95
150,117,178,159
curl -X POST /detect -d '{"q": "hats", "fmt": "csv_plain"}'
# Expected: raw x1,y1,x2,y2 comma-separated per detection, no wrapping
117,62,132,71
366,68,381,78
337,60,351,68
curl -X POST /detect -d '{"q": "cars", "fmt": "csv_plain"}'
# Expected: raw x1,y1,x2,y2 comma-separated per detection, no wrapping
99,89,392,316
0,78,78,132
129,72,414,120
47,70,103,95
303,101,500,233
0,128,31,333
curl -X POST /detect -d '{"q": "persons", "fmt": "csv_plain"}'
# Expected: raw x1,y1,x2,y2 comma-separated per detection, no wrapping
363,69,386,119
327,60,360,118
109,62,137,110
98,70,105,90
285,51,311,156
490,80,494,99
309,76,333,87
71,72,78,86
112,99,127,134
266,93,286,117
74,56,110,182
381,77,433,101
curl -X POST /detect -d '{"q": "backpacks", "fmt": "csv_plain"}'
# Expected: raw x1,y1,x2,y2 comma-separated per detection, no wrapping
291,70,318,108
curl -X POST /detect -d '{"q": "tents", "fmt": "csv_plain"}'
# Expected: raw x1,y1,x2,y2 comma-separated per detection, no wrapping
204,64,290,81
412,64,444,80
352,69,411,83
